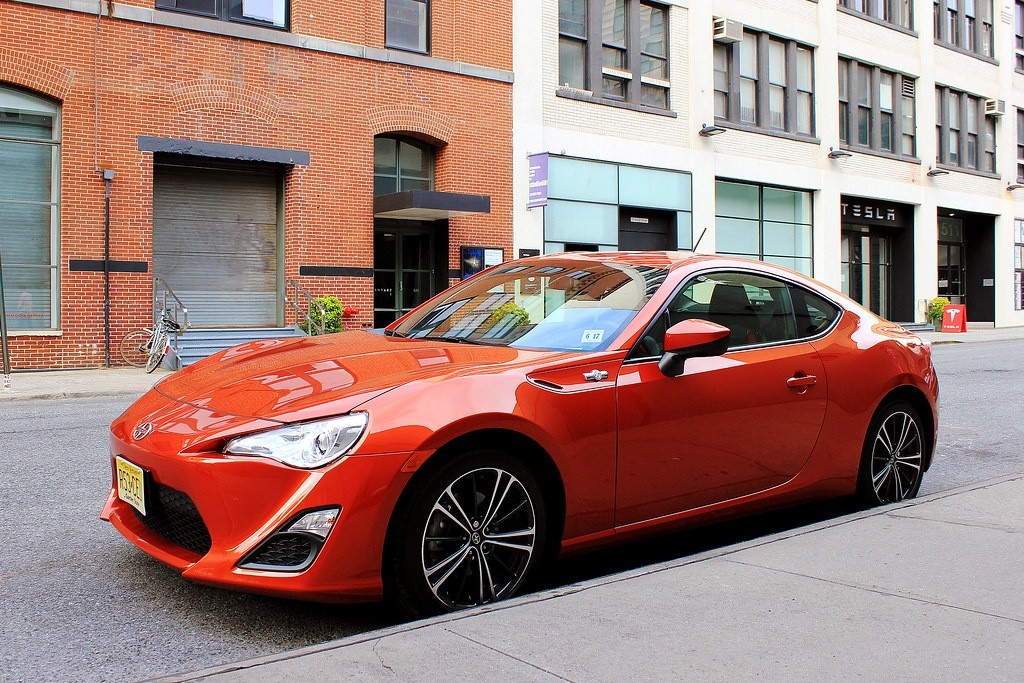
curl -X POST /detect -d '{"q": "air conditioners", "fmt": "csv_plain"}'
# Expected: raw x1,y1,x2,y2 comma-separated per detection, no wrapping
714,17,743,44
985,98,1005,116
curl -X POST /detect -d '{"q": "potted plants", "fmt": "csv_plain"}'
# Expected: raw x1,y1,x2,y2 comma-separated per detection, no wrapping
927,297,950,332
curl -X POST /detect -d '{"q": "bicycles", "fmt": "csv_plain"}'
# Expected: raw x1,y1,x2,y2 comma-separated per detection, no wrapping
120,296,182,375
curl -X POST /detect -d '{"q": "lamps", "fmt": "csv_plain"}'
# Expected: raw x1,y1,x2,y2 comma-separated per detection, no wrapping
828,147,853,160
927,164,949,177
699,123,726,137
1006,181,1024,191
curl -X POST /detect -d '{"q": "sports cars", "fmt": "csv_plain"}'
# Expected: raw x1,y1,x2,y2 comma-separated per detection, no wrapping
98,250,939,619
